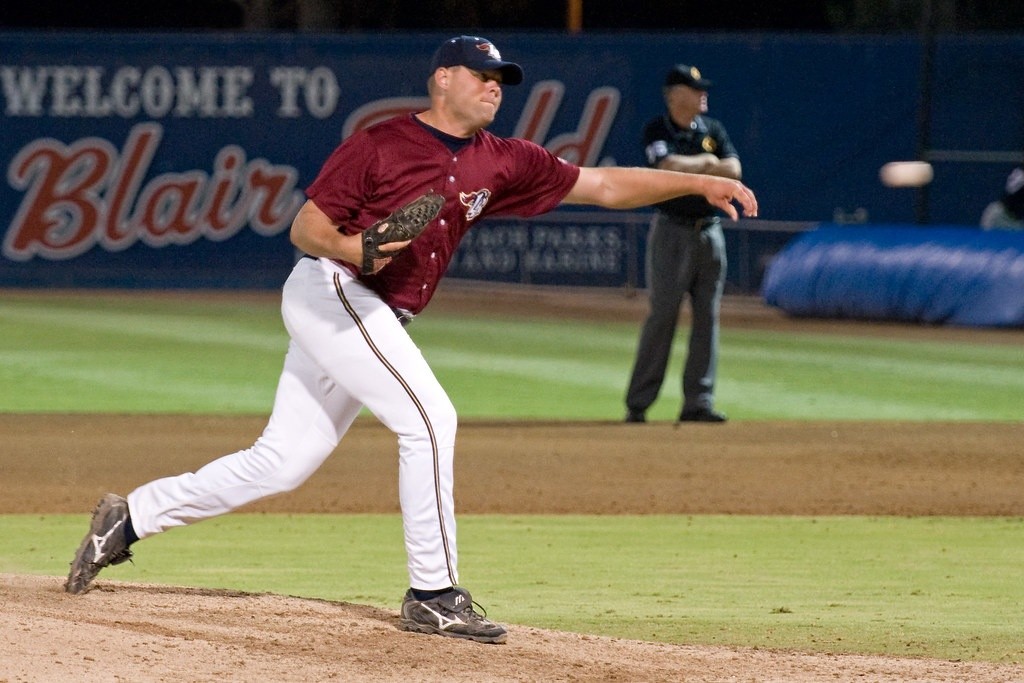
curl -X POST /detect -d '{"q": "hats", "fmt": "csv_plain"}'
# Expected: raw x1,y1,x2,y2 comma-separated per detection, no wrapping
662,64,714,88
427,36,523,85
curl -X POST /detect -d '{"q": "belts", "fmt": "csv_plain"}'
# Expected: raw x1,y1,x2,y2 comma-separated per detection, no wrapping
302,254,411,326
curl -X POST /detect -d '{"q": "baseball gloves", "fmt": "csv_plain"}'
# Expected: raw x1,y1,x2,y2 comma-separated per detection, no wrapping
361,194,451,267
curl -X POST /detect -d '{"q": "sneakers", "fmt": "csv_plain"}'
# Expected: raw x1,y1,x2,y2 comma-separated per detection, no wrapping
400,587,509,645
63,493,134,595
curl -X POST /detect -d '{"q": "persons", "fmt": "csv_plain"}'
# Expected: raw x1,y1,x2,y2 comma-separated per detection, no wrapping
980,167,1024,227
64,36,758,645
622,64,742,423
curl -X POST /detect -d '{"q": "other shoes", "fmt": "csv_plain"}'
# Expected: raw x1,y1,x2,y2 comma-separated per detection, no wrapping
680,405,728,422
626,409,644,423
